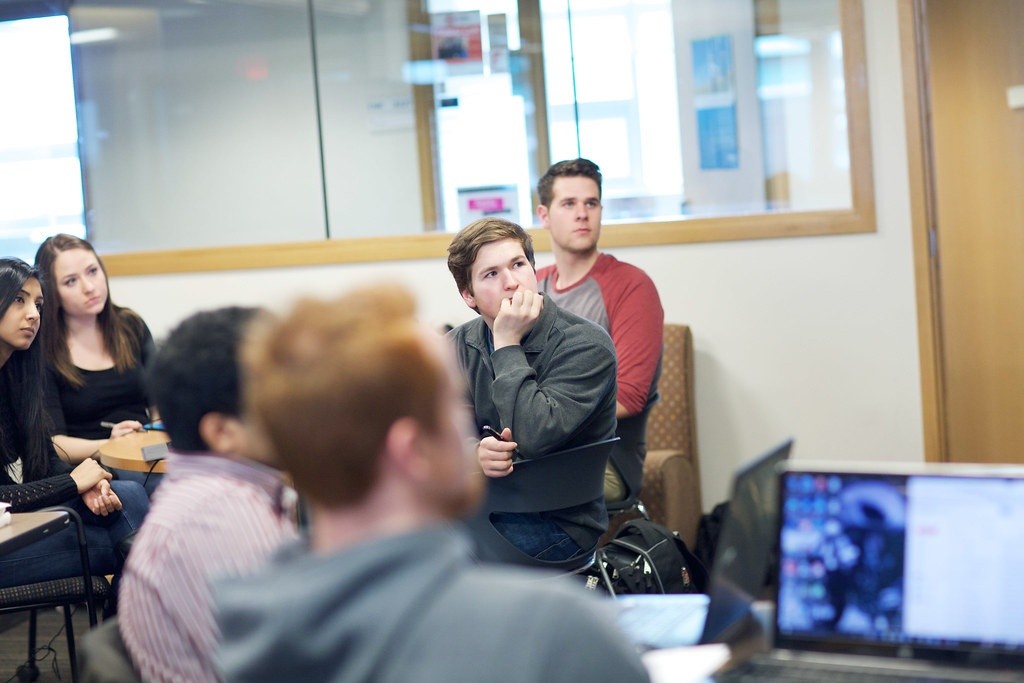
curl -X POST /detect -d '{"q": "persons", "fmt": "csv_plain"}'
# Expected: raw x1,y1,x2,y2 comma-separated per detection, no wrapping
534,159,663,499
441,218,616,576
202,281,652,682
0,254,152,626
111,305,303,682
32,234,166,497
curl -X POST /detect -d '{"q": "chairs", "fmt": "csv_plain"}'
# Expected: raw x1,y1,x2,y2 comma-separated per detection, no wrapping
79,618,141,683
601,393,664,521
0,507,116,682
471,435,623,584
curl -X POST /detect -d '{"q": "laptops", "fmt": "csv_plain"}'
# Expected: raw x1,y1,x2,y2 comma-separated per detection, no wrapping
618,442,793,648
711,462,1024,683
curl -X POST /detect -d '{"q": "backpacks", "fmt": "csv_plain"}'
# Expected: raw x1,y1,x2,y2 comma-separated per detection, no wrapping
596,516,710,595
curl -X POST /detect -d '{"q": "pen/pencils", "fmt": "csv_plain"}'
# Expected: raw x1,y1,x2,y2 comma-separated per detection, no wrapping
483,424,526,457
99,420,119,429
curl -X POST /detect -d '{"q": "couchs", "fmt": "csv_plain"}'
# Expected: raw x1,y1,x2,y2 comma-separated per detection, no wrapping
637,324,706,553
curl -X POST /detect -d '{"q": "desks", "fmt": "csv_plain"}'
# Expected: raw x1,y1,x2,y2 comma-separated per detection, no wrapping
0,511,69,555
99,427,166,475
